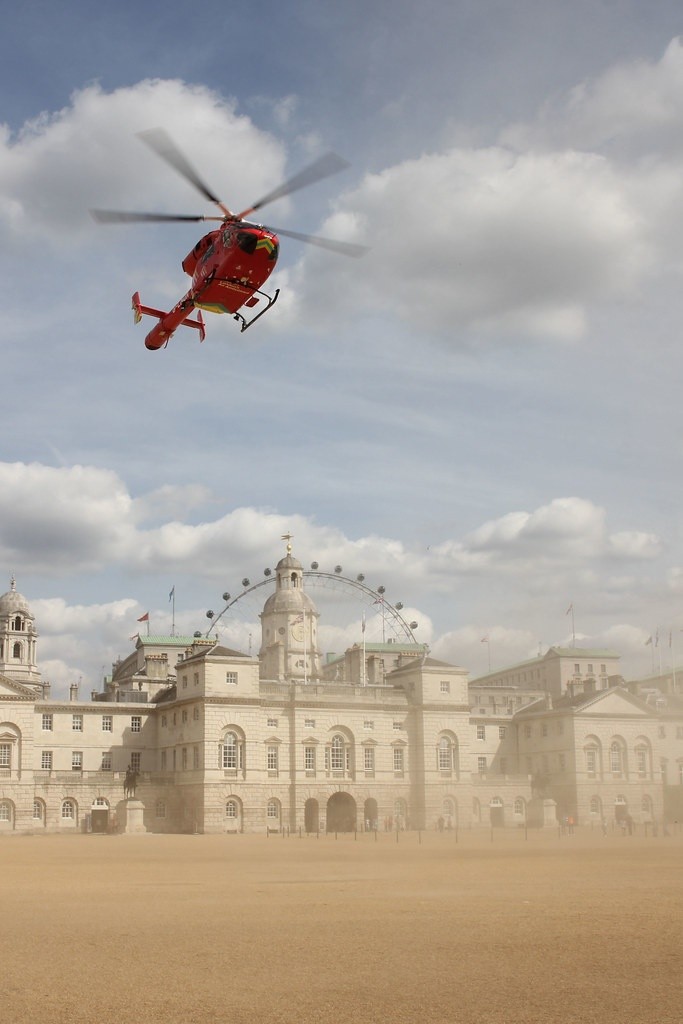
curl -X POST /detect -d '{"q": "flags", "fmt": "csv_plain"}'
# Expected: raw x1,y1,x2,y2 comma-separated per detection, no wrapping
129,633,139,641
373,596,384,605
481,636,488,642
137,612,148,622
169,588,174,602
290,613,303,626
656,632,658,647
645,636,652,645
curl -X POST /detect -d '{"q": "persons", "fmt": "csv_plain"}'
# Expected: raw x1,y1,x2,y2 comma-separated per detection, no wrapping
438,815,445,831
601,817,608,838
562,812,575,836
621,814,632,836
384,814,410,832
366,816,374,831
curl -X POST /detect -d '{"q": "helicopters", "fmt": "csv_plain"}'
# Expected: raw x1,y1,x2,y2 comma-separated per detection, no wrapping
89,126,374,352
